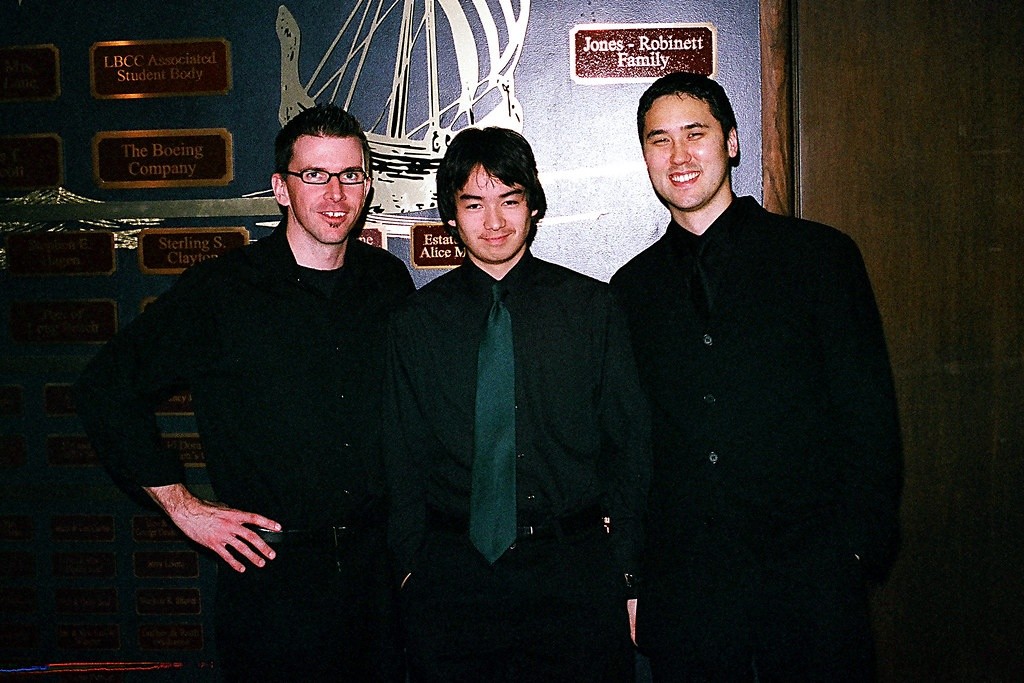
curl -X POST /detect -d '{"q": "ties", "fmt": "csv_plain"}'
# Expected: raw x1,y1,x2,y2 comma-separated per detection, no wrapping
469,283,516,563
688,237,716,326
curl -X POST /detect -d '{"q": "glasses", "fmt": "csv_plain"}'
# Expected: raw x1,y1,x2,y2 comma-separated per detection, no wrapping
284,170,370,185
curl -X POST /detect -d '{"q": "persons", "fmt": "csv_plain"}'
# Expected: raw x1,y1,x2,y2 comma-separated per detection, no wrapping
377,126,654,683
609,71,903,683
74,104,421,683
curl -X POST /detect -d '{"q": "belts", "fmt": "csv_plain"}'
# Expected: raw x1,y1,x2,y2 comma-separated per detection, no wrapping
252,519,384,547
429,504,602,550
